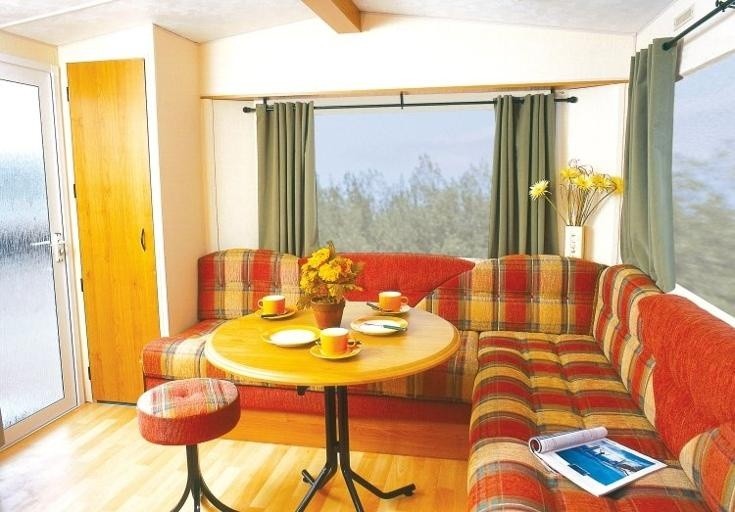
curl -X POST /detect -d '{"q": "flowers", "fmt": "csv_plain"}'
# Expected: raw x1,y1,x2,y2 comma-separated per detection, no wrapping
294,240,365,309
526,157,625,226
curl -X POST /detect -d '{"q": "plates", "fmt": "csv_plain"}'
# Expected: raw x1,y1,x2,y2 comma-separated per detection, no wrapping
351,314,409,337
310,341,363,359
260,325,320,347
364,304,410,315
260,308,295,319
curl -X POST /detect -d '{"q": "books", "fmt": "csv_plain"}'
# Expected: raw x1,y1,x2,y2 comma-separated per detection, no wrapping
529,426,668,499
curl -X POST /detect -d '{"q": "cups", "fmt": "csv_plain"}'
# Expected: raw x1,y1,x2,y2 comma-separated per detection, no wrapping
257,295,285,314
318,327,357,355
378,291,409,311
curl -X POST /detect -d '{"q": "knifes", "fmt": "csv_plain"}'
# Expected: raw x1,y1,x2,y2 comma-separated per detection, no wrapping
363,322,406,332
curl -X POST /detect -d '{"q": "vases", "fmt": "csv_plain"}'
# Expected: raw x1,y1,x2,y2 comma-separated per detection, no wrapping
309,300,345,329
563,224,585,260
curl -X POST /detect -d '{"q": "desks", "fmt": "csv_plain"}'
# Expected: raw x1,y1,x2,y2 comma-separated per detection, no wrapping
203,302,463,512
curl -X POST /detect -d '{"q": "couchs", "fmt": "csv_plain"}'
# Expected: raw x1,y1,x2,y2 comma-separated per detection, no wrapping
136,245,735,511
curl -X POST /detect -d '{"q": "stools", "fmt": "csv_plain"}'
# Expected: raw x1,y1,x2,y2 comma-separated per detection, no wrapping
136,378,242,512
